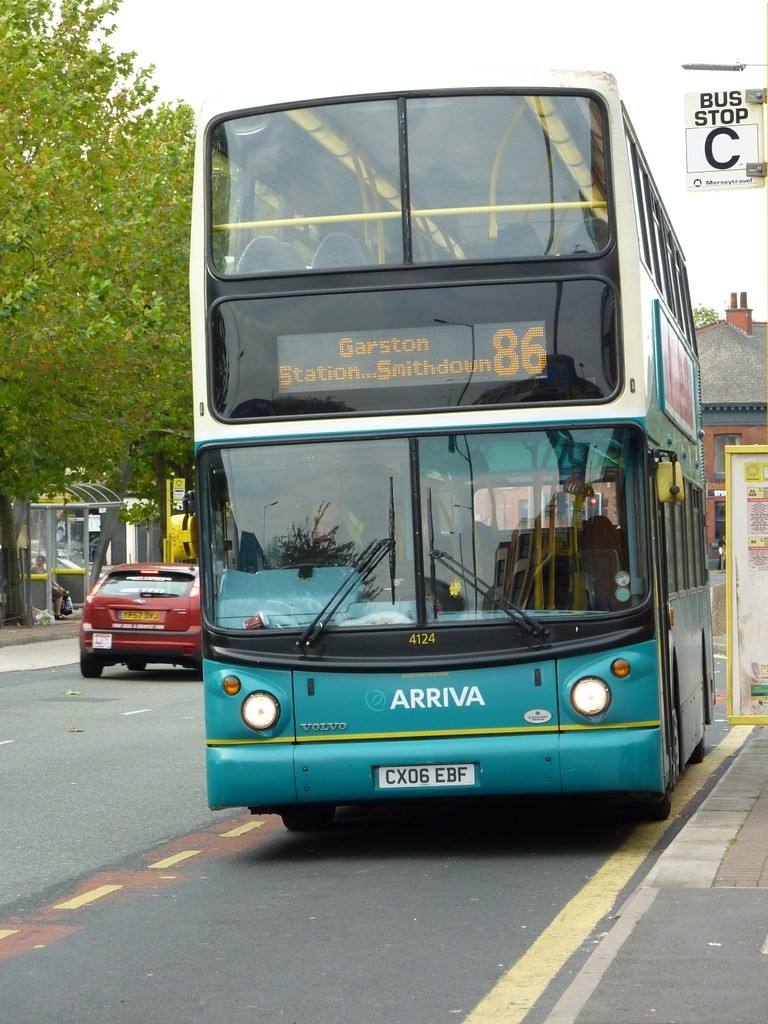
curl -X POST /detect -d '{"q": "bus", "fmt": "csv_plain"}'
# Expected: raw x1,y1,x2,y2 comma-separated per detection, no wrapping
153,69,718,831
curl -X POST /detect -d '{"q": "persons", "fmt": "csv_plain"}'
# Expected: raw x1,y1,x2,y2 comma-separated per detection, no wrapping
57,526,66,543
31,556,66,620
274,499,370,570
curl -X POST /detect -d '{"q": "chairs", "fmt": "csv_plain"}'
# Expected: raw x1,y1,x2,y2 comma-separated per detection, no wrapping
426,516,623,617
238,217,609,277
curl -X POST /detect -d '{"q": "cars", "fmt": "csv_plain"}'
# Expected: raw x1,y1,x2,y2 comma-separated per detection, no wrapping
31,550,82,576
78,562,203,680
89,537,107,565
